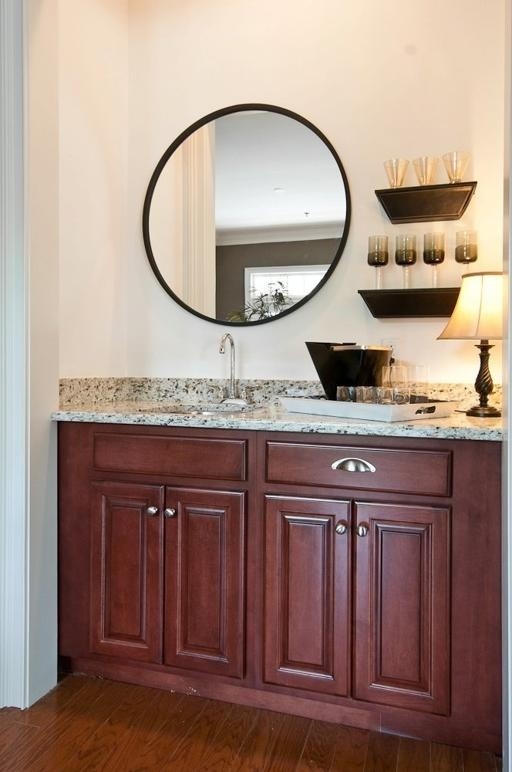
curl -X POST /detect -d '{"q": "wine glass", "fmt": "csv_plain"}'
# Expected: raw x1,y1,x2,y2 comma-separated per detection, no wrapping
423,233,445,287
394,234,416,289
367,235,388,290
454,230,478,275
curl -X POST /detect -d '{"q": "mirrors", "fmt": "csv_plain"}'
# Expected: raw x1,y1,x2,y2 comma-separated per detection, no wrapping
141,99,355,327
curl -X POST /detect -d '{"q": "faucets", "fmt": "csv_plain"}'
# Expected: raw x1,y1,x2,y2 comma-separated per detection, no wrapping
219,332,248,406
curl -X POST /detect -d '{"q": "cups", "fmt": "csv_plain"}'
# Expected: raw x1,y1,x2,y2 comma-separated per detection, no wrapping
336,366,428,415
411,156,440,186
442,151,469,183
382,159,409,187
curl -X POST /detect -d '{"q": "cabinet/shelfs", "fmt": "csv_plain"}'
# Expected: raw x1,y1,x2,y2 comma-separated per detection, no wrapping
50,413,256,712
254,432,502,755
355,180,486,321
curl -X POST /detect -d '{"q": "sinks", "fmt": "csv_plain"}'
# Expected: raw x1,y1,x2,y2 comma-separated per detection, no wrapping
138,403,265,417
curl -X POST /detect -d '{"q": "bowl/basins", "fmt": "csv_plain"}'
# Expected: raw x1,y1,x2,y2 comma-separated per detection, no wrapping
304,342,393,400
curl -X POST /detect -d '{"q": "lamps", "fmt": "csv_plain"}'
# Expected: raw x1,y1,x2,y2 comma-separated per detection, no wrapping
436,270,510,420
